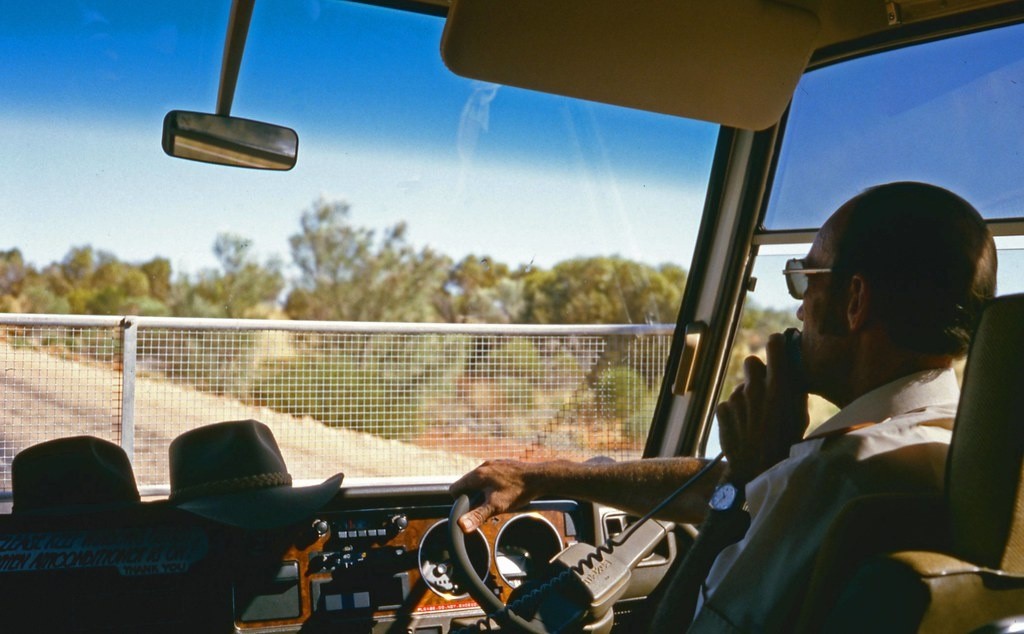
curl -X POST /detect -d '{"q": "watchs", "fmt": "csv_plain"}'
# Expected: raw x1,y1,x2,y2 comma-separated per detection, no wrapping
708,483,749,514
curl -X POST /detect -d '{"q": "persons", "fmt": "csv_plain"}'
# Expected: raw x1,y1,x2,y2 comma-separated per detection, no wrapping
448,181,998,634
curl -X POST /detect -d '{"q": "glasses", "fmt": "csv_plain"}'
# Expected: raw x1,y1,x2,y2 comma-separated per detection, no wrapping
785,258,878,301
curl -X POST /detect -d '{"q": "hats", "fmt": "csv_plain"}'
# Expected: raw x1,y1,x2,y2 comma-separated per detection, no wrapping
0,436,170,527
161,420,344,532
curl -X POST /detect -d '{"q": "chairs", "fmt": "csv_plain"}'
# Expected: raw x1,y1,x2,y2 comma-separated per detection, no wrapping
865,294,1024,634
